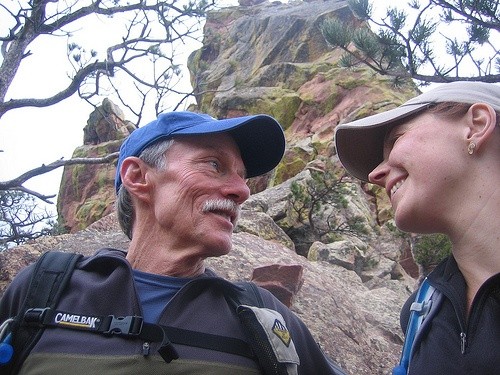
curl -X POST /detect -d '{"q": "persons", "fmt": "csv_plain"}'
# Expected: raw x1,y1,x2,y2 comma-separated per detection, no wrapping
334,81,500,375
0,111,333,375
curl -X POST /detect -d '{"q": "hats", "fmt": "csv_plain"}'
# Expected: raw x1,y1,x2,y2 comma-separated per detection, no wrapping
334,81,500,183
115,111,286,196
